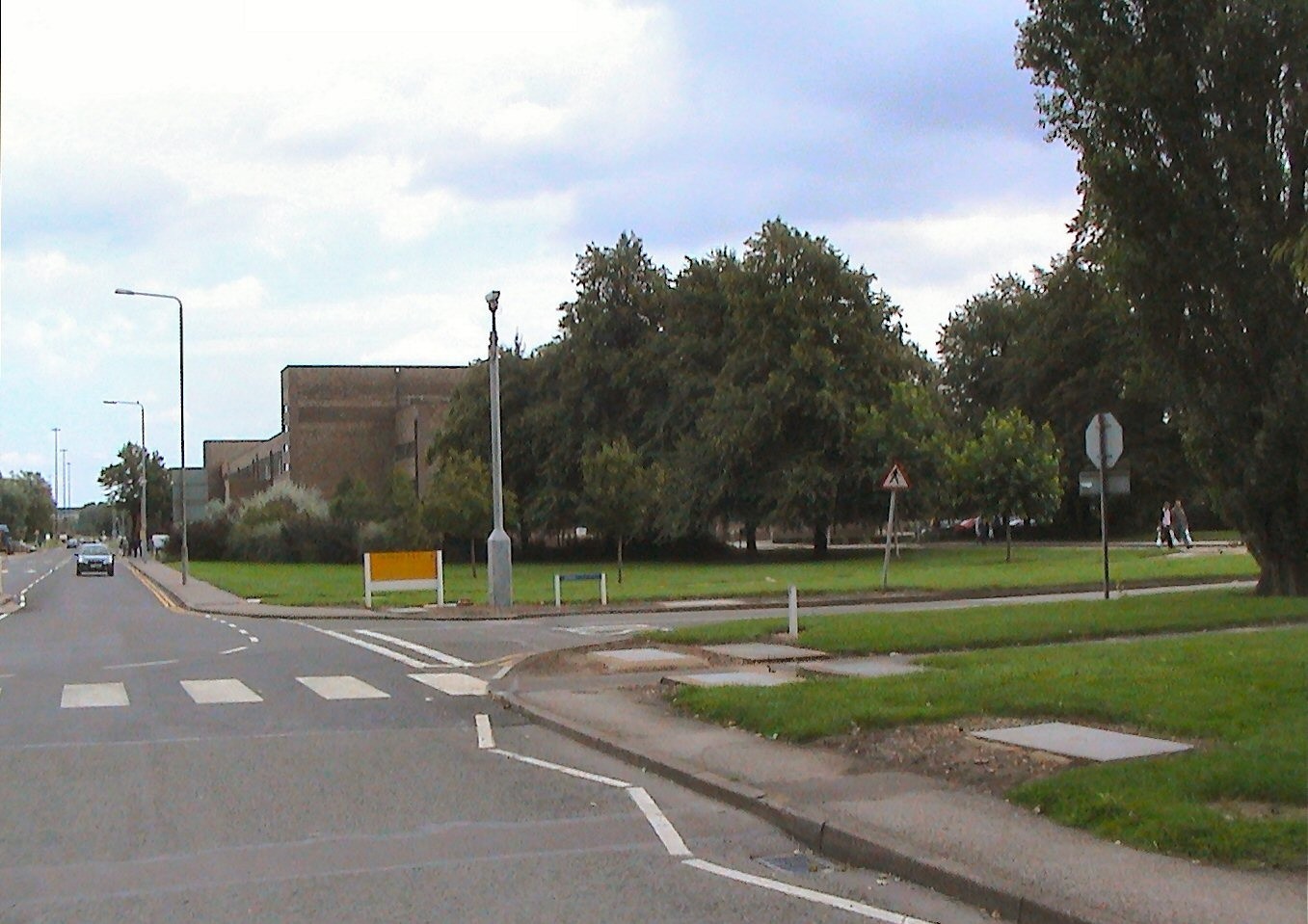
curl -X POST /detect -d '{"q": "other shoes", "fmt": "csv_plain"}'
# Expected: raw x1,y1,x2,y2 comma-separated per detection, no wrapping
1186,545,1193,549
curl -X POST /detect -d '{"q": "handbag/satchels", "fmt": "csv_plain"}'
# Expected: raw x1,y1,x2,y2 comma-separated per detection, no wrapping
1156,531,1163,547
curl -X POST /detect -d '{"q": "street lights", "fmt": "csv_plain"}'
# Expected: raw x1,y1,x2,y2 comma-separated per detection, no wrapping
59,449,68,511
485,290,513,609
66,462,72,513
52,428,62,539
114,288,188,585
103,400,148,564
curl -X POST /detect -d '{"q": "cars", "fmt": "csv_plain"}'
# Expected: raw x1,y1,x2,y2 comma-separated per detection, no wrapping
66,538,78,548
936,514,1038,531
78,535,103,544
74,543,116,577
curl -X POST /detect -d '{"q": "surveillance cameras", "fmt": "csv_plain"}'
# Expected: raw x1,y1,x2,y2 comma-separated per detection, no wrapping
485,290,500,302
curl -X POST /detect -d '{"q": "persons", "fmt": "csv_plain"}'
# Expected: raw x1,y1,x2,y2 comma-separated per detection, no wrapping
121,538,139,558
1154,499,1195,549
